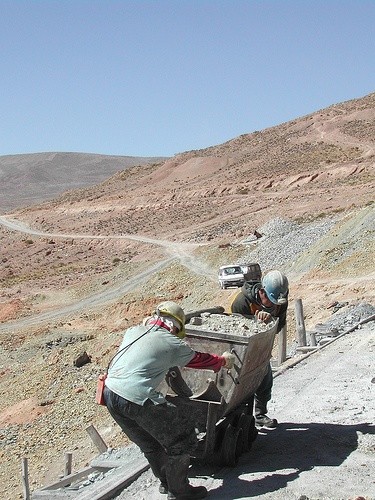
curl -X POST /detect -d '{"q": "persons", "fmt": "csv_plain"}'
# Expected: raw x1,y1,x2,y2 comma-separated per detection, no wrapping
225,270,289,428
102,301,235,499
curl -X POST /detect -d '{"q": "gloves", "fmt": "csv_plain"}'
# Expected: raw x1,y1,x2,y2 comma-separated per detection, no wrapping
222,351,235,370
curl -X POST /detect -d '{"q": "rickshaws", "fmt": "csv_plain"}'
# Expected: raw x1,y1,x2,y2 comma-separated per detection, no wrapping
162,305,280,467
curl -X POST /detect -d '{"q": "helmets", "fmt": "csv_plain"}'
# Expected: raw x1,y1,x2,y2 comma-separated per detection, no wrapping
260,270,289,305
153,300,185,338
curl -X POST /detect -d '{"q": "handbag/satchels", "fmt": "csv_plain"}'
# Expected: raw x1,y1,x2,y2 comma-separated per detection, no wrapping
96,373,107,406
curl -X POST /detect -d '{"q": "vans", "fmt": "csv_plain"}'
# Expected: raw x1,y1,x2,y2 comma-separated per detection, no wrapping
218,262,262,289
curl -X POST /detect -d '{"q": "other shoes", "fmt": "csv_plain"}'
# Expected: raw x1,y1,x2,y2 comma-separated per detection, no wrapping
255,415,277,426
167,485,207,500
